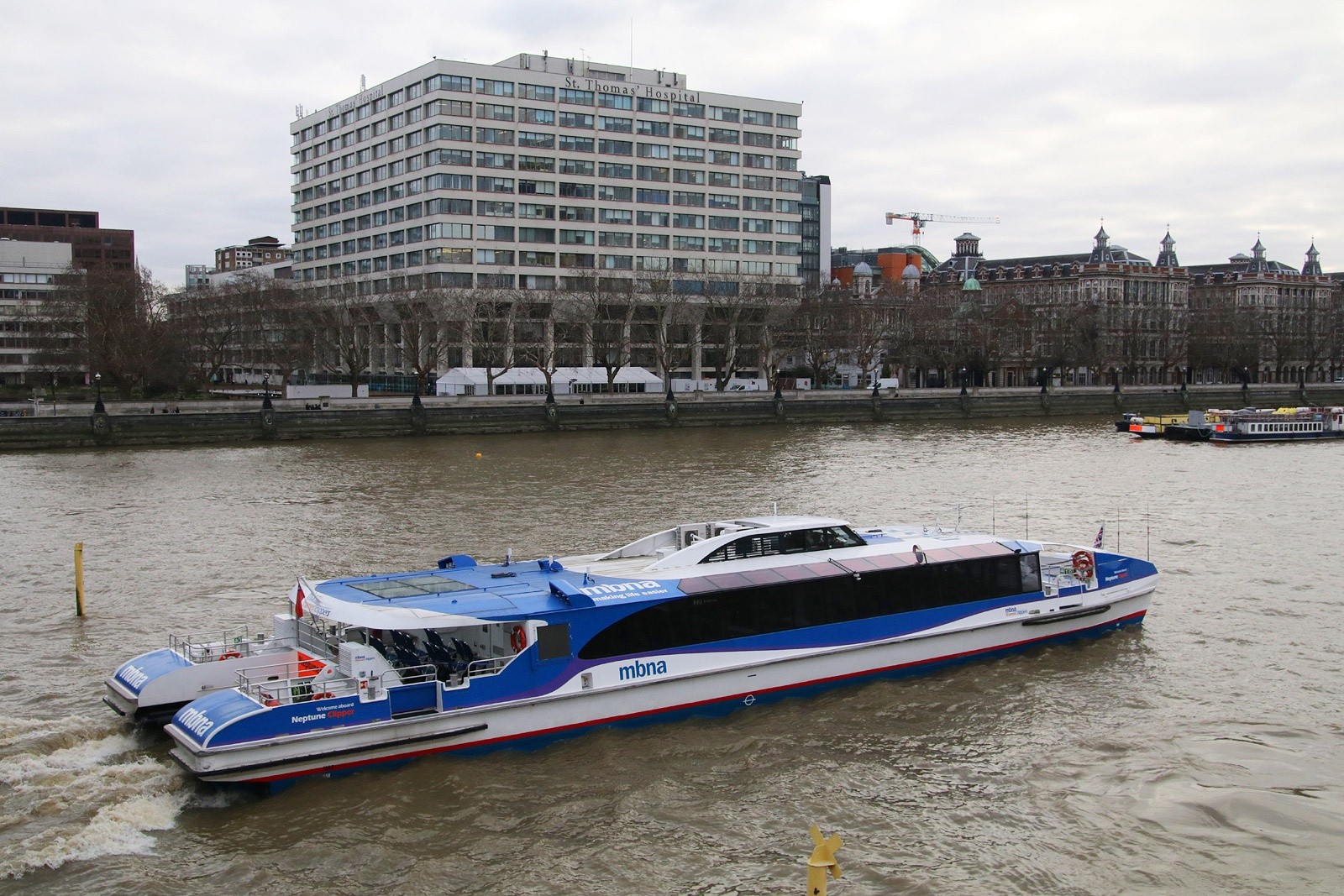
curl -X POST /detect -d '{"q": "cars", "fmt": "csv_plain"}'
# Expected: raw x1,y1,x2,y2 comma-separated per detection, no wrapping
1333,377,1344,383
729,384,752,391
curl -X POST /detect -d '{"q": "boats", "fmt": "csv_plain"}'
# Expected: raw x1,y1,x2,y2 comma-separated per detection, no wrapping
100,501,1159,799
1114,405,1343,444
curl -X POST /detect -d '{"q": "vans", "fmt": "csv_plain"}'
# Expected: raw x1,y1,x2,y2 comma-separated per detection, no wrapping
866,378,899,390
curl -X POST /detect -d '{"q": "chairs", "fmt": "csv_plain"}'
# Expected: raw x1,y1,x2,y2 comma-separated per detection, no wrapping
358,628,490,683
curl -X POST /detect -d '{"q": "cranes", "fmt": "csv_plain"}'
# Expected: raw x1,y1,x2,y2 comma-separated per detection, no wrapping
885,212,1000,248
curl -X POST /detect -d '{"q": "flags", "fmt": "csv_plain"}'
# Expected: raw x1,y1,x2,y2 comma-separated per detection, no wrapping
1093,523,1103,549
295,582,305,619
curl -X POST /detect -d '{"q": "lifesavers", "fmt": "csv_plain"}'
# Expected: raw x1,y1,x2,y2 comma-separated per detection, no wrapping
220,649,243,660
1333,415,1337,421
510,625,527,653
313,692,336,700
1072,550,1092,580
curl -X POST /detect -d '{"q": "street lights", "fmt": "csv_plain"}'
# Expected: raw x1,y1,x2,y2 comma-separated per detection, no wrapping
94,372,106,413
774,369,783,398
1112,367,1121,392
1242,367,1248,390
262,372,274,409
960,367,968,395
1299,366,1305,389
412,371,423,406
1040,367,1048,393
666,368,676,401
427,342,437,395
871,369,880,397
1180,367,1187,391
